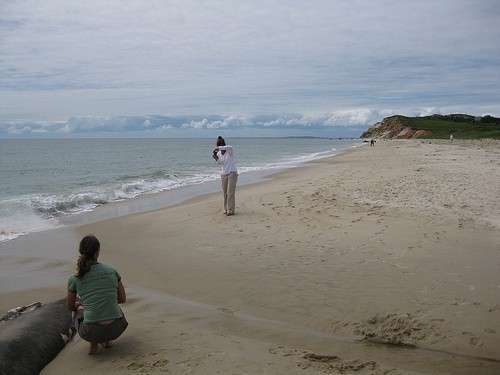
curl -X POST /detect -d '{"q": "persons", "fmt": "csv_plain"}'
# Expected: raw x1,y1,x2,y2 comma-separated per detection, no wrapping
212,136,237,216
450,134,454,144
370,139,374,147
67,234,128,355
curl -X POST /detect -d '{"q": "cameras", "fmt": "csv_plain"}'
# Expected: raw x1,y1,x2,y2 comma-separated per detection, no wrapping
214,152,217,155
